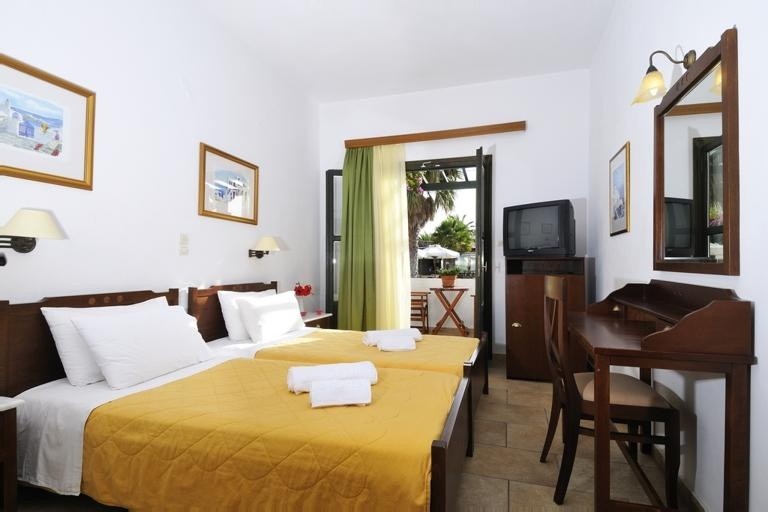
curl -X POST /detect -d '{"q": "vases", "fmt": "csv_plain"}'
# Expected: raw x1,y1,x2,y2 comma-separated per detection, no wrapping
296,295,306,314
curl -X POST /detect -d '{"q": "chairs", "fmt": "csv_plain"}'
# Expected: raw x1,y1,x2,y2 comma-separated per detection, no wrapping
410,292,428,331
540,275,682,506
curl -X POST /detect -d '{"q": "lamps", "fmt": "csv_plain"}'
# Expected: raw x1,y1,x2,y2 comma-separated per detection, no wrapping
629,44,698,109
249,236,280,259
0,206,68,267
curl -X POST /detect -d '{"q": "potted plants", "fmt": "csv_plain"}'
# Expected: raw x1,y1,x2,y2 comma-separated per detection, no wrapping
438,266,462,287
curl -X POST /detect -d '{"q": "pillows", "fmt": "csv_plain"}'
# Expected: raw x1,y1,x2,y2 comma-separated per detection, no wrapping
218,289,277,343
40,295,169,388
236,291,305,343
69,308,215,391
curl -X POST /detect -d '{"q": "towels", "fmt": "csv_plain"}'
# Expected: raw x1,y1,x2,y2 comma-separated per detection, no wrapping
309,378,374,407
285,360,378,395
363,328,422,347
376,334,417,353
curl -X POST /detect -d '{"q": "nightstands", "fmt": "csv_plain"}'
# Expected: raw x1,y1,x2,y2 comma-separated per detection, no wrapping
302,308,333,329
0,398,19,512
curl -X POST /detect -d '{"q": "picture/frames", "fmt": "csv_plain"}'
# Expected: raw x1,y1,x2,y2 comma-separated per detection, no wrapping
608,141,630,237
198,141,260,227
0,53,96,192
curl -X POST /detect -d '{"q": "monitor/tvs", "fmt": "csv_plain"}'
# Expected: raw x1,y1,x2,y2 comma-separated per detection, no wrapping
502,199,577,255
663,197,696,257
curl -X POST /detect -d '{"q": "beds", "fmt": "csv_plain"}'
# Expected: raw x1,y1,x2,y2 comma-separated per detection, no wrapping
189,281,489,444
0,288,475,512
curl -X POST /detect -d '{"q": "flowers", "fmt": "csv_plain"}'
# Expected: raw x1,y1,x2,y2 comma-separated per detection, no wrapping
295,282,312,296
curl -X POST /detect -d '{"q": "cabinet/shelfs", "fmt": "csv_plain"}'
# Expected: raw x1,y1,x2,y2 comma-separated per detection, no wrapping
504,274,587,383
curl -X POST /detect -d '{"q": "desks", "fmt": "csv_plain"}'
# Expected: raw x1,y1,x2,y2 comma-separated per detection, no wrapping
429,287,470,337
567,278,758,511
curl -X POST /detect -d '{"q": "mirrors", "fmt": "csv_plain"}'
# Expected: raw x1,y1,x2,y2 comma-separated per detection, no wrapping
649,28,744,279
